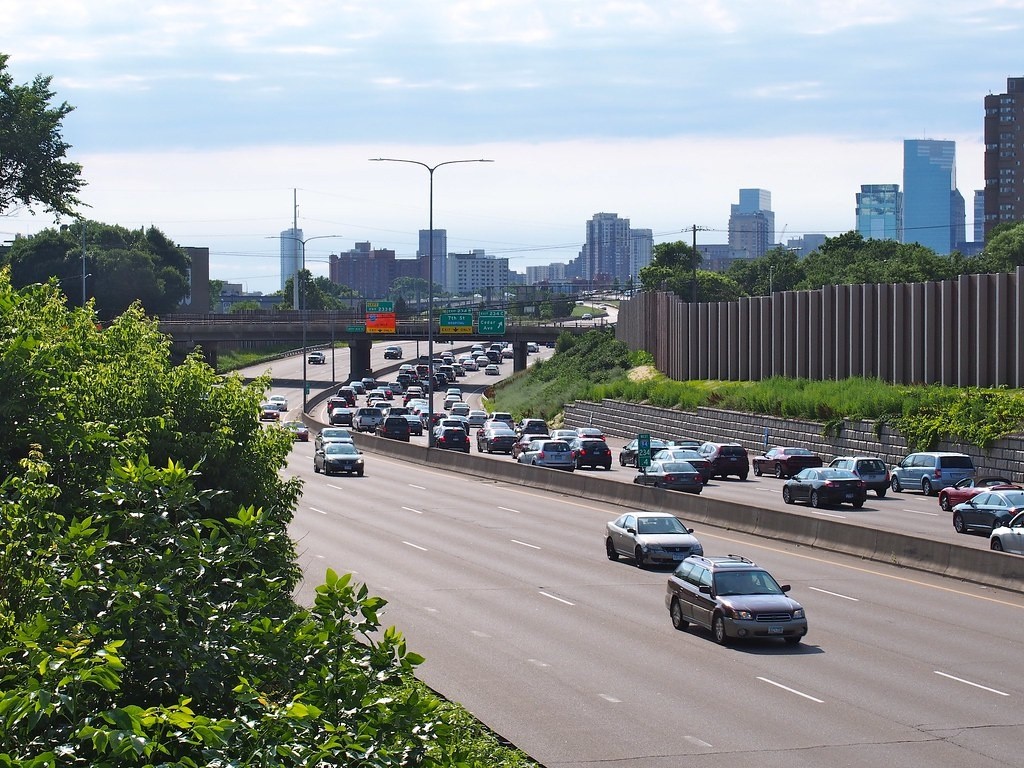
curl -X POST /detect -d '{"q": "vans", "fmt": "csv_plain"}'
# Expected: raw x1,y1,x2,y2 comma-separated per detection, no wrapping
889,451,976,496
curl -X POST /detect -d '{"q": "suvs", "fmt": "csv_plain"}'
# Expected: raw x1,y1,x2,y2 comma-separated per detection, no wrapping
664,554,808,647
696,442,750,481
828,456,891,498
383,346,402,359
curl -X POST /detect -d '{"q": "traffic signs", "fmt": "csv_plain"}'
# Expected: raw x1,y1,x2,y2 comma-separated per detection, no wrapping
366,312,396,334
478,316,505,335
439,313,474,334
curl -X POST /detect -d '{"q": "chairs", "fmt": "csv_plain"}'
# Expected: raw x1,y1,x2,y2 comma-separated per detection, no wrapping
638,519,671,532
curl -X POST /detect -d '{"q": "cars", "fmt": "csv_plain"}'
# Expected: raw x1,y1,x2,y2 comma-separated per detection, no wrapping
260,405,281,421
619,439,668,469
582,313,593,321
598,303,607,310
283,421,309,442
752,447,823,479
633,461,703,495
952,490,1024,534
667,439,702,450
308,351,325,364
269,395,288,412
782,467,868,508
327,340,606,459
938,476,1023,511
314,443,365,476
569,438,612,471
517,439,575,472
651,449,712,485
315,428,355,451
989,510,1024,556
604,511,703,569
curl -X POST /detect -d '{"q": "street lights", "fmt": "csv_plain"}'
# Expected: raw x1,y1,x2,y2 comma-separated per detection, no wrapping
366,158,495,448
305,259,357,386
83,273,92,307
265,234,343,413
